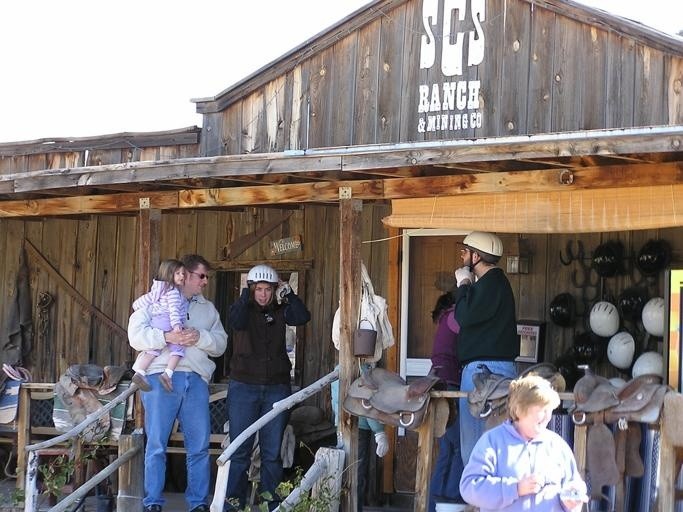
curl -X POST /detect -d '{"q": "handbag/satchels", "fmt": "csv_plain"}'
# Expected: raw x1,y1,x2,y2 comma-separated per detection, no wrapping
66,364,104,388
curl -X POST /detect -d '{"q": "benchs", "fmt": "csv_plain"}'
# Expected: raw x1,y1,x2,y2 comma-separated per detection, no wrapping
167,391,258,505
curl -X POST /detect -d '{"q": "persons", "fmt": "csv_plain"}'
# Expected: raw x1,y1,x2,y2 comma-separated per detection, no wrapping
221,264,312,512
330,363,389,511
127,254,229,512
453,230,520,468
459,376,589,512
426,282,464,512
127,259,185,392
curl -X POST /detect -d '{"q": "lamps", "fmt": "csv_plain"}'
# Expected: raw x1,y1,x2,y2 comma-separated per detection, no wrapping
506,240,530,274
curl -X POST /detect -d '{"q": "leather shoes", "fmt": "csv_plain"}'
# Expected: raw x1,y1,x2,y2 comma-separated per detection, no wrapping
145,504,161,512
190,504,210,512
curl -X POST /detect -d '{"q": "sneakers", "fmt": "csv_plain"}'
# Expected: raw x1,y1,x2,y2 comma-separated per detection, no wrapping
132,371,152,391
158,372,173,392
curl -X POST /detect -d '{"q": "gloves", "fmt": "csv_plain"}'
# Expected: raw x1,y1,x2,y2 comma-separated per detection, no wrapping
374,432,389,457
275,279,292,304
455,266,474,288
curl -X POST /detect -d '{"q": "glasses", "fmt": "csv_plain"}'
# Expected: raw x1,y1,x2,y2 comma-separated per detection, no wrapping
188,270,208,279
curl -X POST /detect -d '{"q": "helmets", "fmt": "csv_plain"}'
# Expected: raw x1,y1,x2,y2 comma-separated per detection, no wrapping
246,265,279,289
549,240,671,379
457,231,503,264
